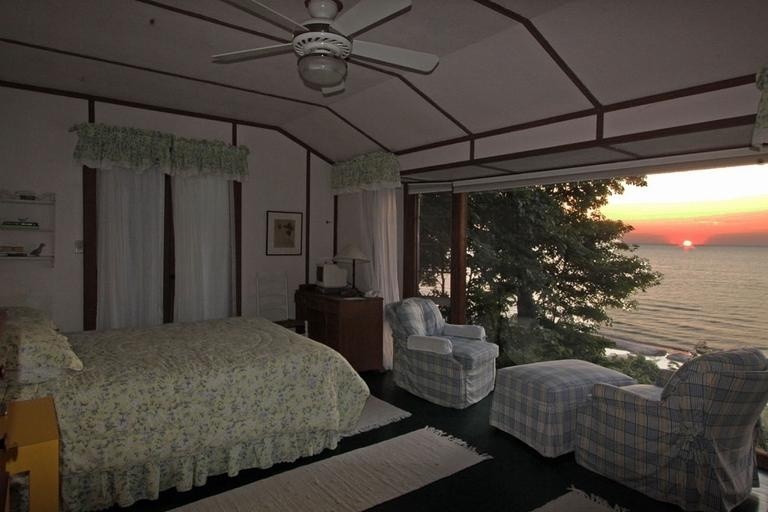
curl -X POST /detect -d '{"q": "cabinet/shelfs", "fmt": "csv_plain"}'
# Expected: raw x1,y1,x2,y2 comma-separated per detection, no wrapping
0,191,55,268
293,290,384,367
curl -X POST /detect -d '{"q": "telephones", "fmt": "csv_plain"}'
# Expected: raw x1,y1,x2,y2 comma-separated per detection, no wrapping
365,288,378,298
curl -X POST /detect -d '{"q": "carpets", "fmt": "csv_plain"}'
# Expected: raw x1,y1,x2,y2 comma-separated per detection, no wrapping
175,425,494,512
532,486,630,512
343,396,410,438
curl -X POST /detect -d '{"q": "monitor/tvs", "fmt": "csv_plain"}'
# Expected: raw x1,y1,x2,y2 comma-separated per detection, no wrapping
315,264,347,288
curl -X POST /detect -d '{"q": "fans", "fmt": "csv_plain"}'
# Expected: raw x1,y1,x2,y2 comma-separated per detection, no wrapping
210,0,439,96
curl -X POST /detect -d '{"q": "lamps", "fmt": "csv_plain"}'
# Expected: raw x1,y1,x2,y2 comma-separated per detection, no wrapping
333,244,371,296
297,53,348,86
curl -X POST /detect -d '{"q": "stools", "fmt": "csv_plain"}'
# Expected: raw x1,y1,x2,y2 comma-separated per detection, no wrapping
490,358,639,459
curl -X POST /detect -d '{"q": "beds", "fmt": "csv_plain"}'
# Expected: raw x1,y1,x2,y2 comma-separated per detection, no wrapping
0,319,369,512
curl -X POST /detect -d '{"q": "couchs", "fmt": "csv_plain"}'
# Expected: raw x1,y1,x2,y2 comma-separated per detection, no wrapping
384,297,499,410
574,346,768,511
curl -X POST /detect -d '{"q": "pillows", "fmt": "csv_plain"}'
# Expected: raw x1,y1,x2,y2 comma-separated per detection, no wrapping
1,306,83,384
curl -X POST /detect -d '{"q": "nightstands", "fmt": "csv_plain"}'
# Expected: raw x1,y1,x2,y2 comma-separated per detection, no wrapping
0,397,60,512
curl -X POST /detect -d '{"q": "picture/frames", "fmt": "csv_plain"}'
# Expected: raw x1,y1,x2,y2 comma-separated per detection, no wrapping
264,211,304,256
